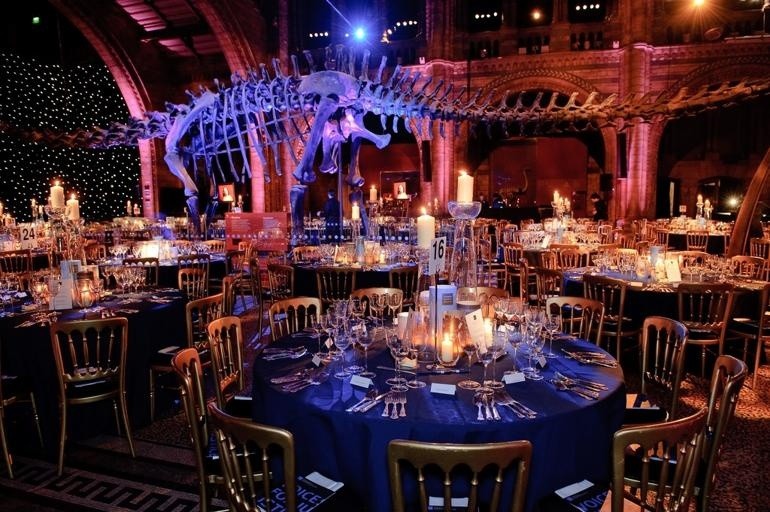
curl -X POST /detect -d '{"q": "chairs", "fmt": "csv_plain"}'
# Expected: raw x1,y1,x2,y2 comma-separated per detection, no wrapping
0,216,770,510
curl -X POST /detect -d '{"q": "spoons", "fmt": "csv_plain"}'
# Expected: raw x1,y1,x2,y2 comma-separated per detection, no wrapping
547,378,597,399
290,371,329,393
472,392,486,422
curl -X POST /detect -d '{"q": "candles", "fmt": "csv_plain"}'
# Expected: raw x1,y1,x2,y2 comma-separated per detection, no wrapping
66,194,79,219
554,191,570,208
698,194,711,208
456,168,472,202
50,179,63,207
416,206,436,247
370,185,377,200
352,202,360,219
32,198,44,212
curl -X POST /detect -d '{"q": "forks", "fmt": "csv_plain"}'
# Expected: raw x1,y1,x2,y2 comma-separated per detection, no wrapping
381,392,407,421
259,346,308,362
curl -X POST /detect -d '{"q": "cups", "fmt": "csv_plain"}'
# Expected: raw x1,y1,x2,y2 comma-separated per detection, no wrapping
3,240,13,252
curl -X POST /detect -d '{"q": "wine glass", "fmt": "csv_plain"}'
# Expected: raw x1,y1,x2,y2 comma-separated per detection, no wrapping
316,241,413,267
0,263,147,317
107,243,213,263
521,224,590,248
590,249,708,285
309,291,559,396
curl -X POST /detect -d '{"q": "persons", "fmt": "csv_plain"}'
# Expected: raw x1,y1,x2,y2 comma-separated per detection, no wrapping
315,190,342,239
590,193,607,222
222,187,233,202
396,184,406,198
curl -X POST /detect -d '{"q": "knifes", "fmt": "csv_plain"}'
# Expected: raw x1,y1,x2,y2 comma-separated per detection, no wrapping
482,393,492,421
494,391,539,420
